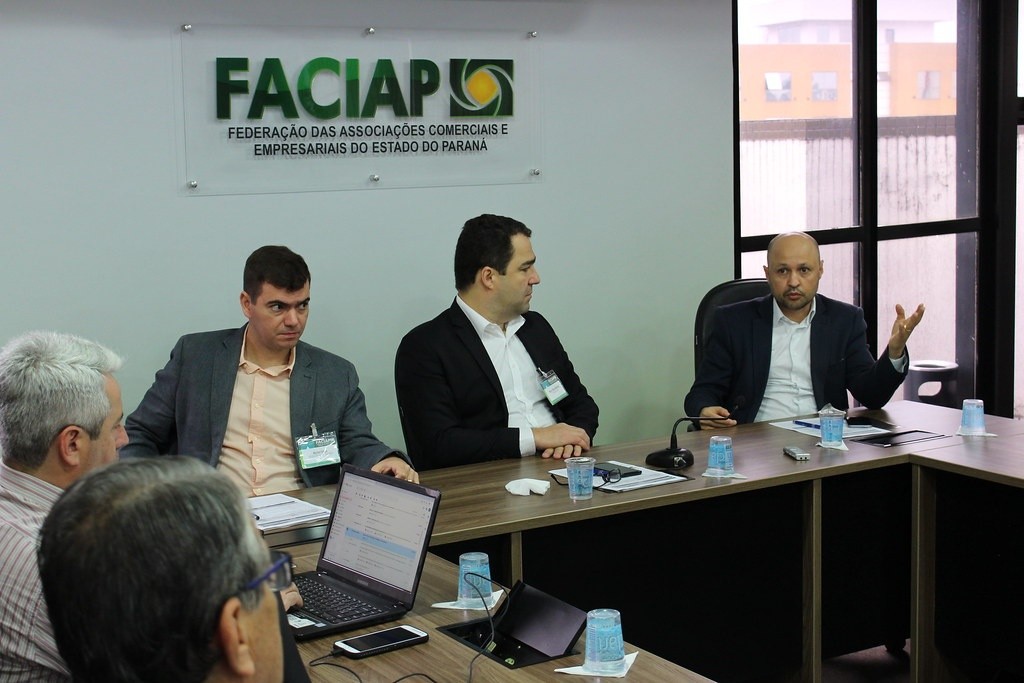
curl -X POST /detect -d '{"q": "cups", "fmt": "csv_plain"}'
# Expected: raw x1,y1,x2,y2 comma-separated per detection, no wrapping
457,552,493,608
701,436,735,477
956,399,986,436
817,403,846,446
564,457,596,500
582,609,626,674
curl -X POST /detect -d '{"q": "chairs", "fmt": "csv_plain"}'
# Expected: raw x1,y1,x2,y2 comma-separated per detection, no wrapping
685,277,772,430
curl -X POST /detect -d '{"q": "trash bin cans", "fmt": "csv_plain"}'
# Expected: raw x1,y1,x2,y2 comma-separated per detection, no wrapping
904,360,960,410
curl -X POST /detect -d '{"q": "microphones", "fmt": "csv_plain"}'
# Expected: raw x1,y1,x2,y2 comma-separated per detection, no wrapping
646,400,745,469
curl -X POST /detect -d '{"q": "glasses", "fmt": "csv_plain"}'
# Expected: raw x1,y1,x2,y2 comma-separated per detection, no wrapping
235,551,291,595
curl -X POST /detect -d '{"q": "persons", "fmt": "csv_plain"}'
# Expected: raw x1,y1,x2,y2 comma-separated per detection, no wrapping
394,213,600,470
685,231,926,652
37,456,284,683
119,246,420,497
0,330,303,683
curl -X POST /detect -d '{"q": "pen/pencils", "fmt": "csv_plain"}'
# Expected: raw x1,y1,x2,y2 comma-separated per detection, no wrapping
792,420,820,430
248,511,260,521
591,468,609,476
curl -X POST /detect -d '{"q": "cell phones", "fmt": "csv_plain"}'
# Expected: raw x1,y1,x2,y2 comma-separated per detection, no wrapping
594,462,642,478
333,624,429,660
847,416,872,428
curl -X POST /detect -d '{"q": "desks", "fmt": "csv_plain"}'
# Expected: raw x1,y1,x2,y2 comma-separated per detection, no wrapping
248,401,1024,683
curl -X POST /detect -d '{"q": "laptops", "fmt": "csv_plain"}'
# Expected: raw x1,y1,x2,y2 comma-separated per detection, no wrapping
283,463,442,642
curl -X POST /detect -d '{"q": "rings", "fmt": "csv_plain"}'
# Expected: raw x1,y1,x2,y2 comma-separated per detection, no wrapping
904,325,906,328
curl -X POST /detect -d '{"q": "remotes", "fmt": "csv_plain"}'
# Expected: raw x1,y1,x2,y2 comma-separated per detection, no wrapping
782,446,811,460
